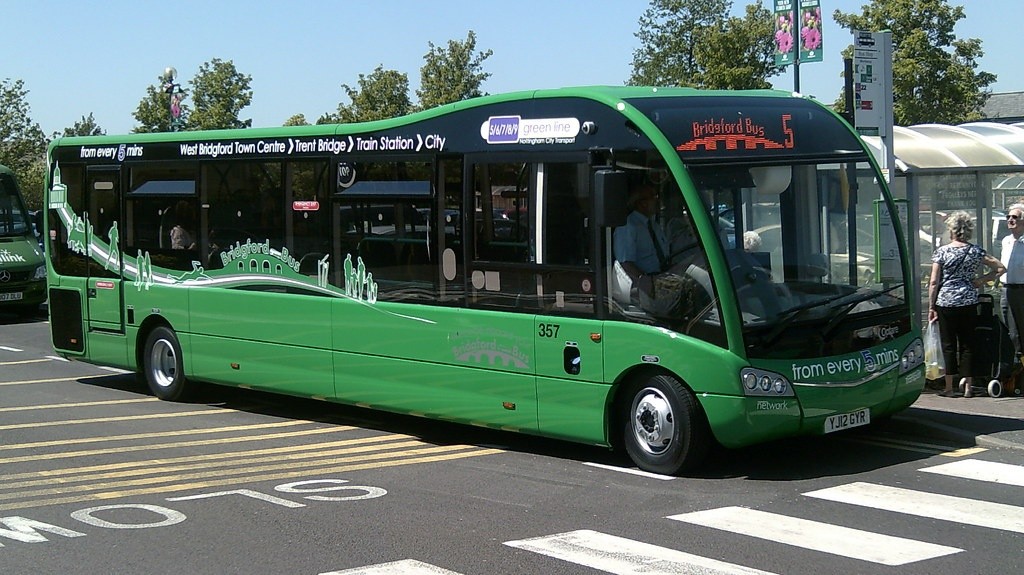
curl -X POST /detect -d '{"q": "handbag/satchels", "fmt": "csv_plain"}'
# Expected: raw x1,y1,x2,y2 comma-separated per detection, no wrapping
923,320,946,380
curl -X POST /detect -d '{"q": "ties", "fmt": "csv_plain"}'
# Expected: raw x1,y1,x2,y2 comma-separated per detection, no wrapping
647,218,665,267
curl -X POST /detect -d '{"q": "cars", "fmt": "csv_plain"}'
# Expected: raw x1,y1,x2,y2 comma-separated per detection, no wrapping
368,203,529,245
710,197,1010,285
0,208,37,236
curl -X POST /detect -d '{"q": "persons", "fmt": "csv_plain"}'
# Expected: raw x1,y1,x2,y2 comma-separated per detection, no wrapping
170,201,219,260
743,230,762,251
928,210,1007,397
667,190,731,277
613,185,670,288
991,202,1024,366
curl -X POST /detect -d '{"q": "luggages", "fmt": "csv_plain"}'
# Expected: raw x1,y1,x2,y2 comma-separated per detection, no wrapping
958,293,1013,398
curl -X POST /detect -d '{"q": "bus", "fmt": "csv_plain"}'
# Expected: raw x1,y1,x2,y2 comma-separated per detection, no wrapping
37,82,927,477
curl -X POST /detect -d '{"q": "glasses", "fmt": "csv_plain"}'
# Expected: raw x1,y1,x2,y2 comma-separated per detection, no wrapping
639,191,659,202
1006,215,1021,220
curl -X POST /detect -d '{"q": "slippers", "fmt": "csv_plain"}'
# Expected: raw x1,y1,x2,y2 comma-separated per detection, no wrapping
939,390,954,398
965,391,975,399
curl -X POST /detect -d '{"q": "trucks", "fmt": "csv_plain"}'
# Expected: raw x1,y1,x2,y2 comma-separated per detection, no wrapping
0,164,48,319
